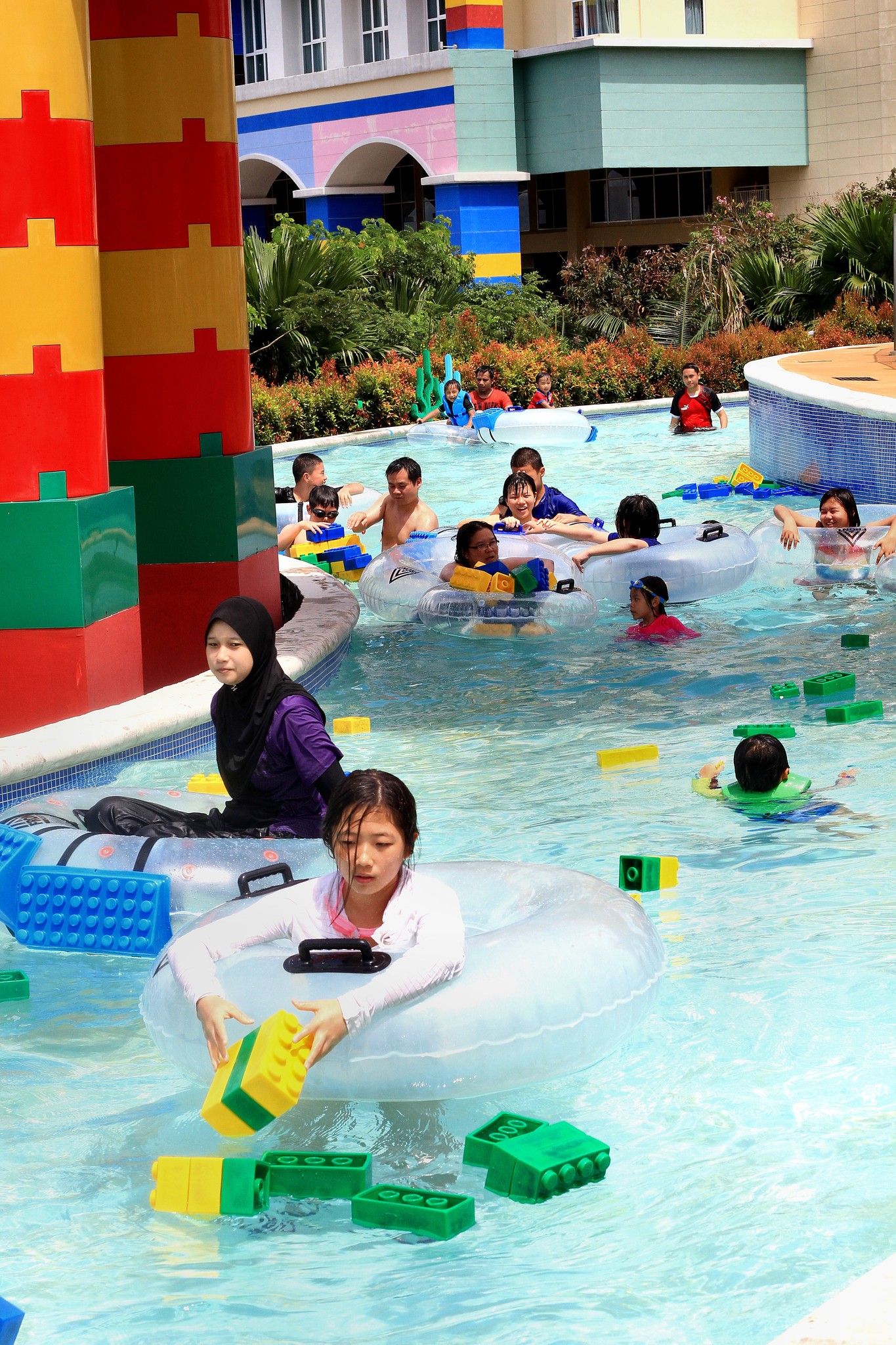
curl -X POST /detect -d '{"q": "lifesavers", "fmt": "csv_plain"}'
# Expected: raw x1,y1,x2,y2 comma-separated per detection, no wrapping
139,859,669,1100
429,518,609,548
477,406,592,445
275,485,384,535
557,518,759,604
358,536,584,622
407,423,483,447
748,504,896,587
418,581,599,639
0,786,338,957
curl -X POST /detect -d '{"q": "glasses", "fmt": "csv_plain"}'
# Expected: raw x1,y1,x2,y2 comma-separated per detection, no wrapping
324,877,400,938
467,540,500,551
310,506,339,519
629,580,666,606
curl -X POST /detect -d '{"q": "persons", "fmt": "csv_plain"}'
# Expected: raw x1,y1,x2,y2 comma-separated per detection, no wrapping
346,456,440,554
522,493,663,574
489,446,594,526
438,520,555,592
624,576,701,645
455,472,590,538
275,452,365,509
526,372,556,410
690,732,891,841
772,487,896,603
163,765,466,1072
277,484,340,557
416,380,476,444
82,594,349,839
669,362,728,435
446,365,515,444
